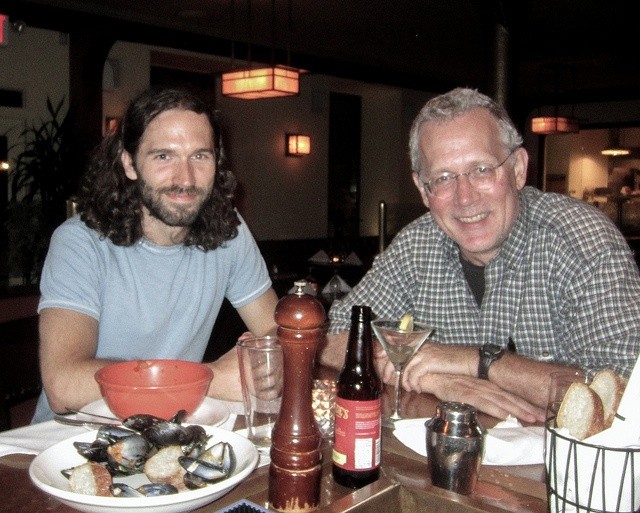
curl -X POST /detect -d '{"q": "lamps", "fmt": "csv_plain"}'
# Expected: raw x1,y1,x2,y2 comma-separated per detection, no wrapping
288,134,312,156
532,116,579,134
222,63,309,100
601,127,631,156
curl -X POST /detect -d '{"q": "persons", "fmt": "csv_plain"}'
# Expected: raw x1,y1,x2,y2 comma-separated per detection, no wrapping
316,86,639,425
604,149,638,188
29,85,280,420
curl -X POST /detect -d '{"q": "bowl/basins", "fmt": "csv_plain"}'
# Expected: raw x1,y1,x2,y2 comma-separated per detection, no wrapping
94,360,215,425
28,425,260,513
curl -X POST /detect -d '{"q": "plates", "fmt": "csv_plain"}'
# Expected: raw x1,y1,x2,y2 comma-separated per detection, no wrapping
79,395,231,429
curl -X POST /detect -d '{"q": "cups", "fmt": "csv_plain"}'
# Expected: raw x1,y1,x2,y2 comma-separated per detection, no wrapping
546,371,595,428
310,377,339,447
236,336,285,448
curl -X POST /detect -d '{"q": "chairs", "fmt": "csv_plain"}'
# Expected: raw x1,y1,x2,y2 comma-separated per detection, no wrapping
595,201,620,225
619,195,640,227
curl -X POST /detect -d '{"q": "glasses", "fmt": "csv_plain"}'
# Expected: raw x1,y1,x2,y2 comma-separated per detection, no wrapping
418,150,513,199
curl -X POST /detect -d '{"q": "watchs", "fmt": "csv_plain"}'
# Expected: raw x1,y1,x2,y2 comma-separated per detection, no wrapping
477,342,503,379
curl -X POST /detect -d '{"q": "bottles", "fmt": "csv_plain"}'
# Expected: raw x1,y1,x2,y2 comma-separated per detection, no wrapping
423,400,483,496
332,304,383,491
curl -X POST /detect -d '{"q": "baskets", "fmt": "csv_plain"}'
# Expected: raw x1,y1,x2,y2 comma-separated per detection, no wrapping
543,413,640,513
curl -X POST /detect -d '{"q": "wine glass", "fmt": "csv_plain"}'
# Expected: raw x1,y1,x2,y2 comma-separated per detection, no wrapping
370,319,436,428
